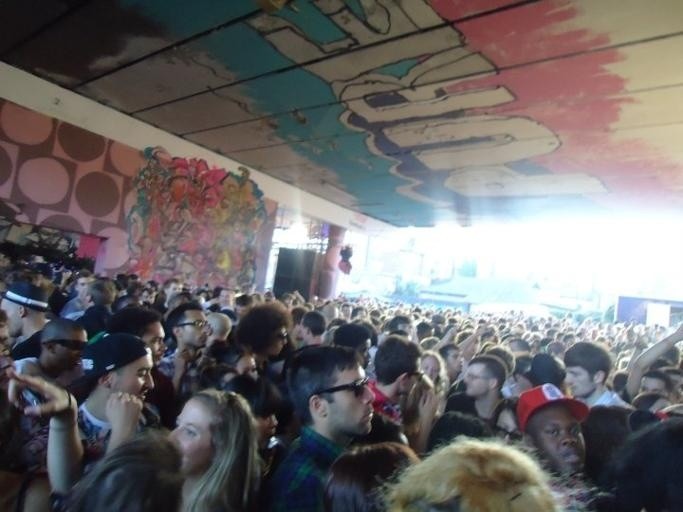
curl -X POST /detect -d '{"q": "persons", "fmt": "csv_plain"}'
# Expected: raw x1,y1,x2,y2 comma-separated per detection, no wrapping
0,252,682,511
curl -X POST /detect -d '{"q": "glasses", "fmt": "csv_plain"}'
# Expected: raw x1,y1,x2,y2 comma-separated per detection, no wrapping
466,372,495,381
46,338,88,353
175,320,209,328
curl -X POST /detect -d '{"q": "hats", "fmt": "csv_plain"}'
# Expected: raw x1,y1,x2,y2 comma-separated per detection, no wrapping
305,374,372,405
1,281,50,313
78,332,152,382
512,383,592,433
